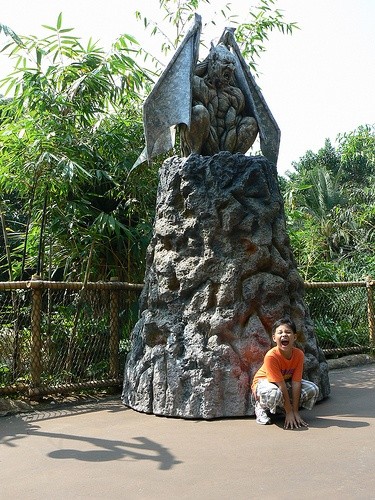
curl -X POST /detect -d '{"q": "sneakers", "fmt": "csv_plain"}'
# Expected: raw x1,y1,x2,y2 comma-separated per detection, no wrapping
279,406,286,416
253,400,272,425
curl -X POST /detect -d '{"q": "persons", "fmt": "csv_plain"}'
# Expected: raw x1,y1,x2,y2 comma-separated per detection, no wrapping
249,317,320,430
